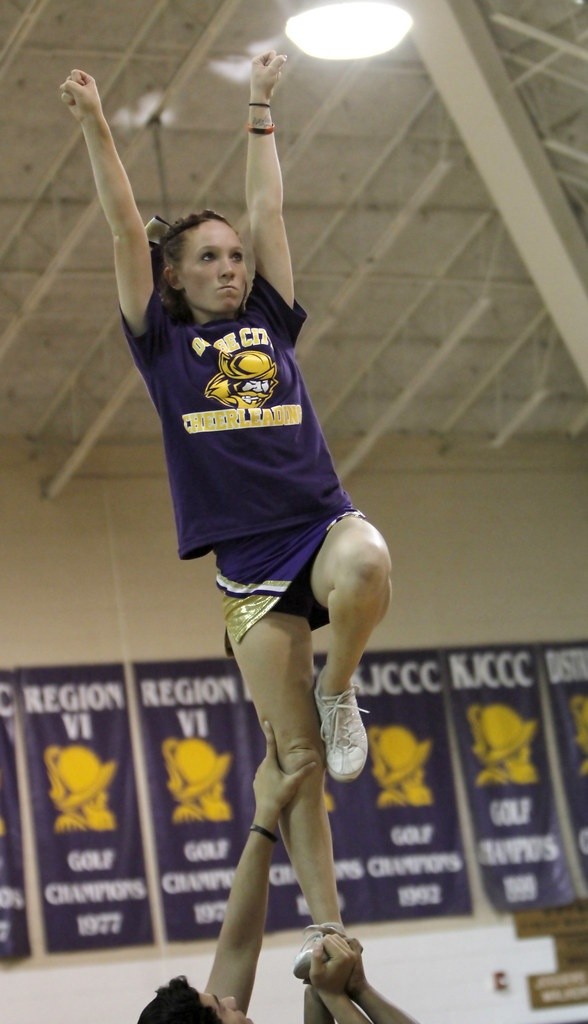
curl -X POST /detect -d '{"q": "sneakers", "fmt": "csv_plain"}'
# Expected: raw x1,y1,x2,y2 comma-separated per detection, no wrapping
314,663,369,785
293,922,348,979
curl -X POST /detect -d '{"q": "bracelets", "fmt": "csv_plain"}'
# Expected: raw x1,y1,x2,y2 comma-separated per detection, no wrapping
249,103,271,107
250,824,279,843
246,122,275,135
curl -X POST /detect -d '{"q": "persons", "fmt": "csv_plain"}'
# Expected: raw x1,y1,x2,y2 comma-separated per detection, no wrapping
309,934,418,1024
138,721,335,1024
57,50,393,983
